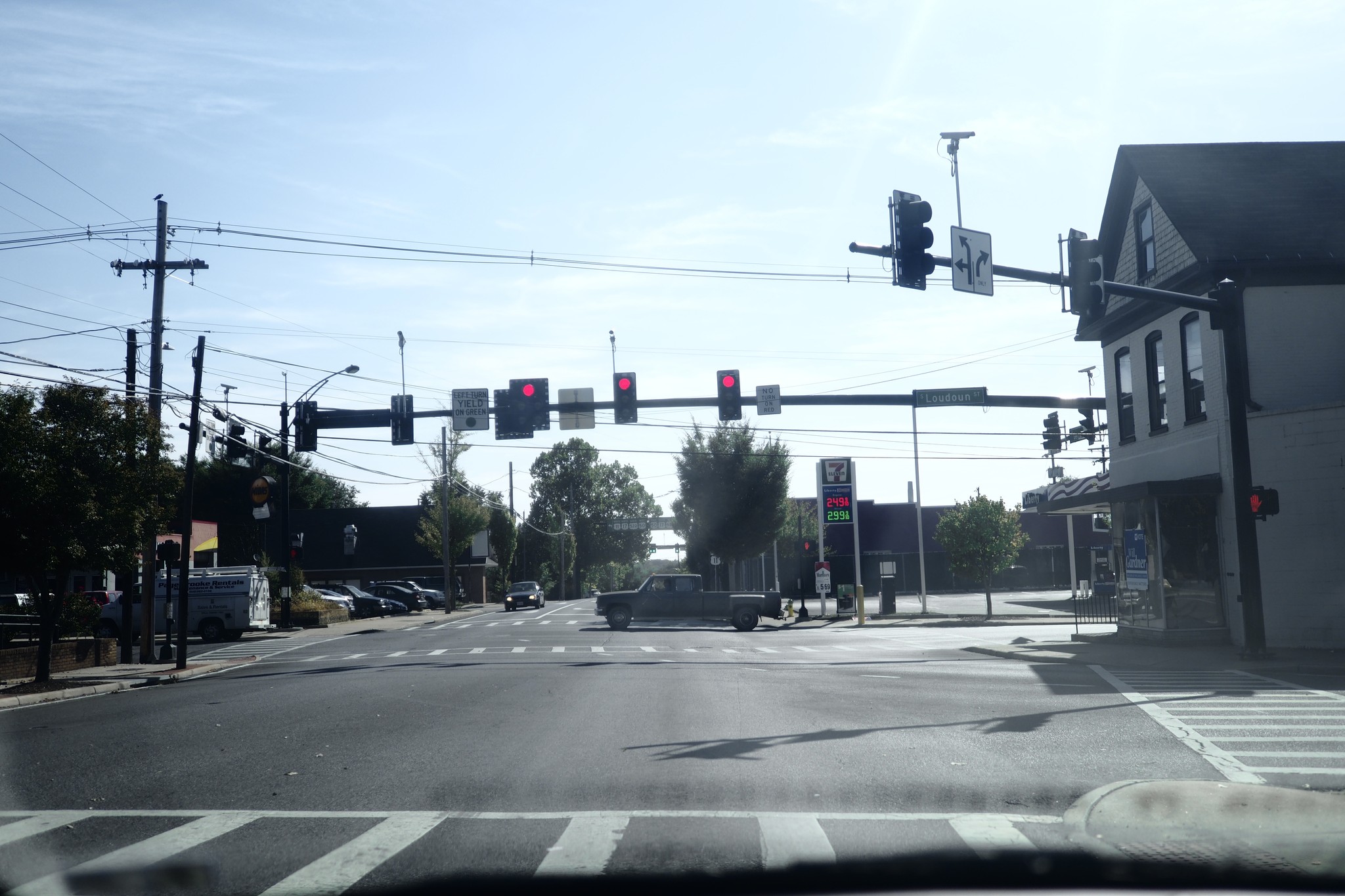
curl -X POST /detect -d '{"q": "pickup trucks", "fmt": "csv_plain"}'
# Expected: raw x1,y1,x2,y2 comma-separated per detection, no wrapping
595,573,786,632
400,576,466,600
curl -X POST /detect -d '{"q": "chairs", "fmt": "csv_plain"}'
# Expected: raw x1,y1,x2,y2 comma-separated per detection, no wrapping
515,588,520,592
519,587,524,591
383,590,388,594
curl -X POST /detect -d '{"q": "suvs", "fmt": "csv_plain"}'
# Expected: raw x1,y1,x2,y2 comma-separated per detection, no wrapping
296,583,393,619
369,580,445,610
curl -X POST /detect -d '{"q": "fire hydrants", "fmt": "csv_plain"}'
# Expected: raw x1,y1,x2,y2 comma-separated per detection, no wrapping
785,598,794,617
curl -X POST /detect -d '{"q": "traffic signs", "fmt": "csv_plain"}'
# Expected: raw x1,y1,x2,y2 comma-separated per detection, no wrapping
950,226,993,297
606,518,646,533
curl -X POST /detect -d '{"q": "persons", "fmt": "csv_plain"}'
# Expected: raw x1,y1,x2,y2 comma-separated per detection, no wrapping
651,579,671,592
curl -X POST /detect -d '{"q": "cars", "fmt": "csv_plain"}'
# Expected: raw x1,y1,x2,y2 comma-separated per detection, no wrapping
360,585,427,612
504,582,544,611
584,589,601,598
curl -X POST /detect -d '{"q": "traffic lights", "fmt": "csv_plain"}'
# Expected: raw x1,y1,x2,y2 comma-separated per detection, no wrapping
896,199,935,281
717,369,742,421
613,372,637,424
228,418,247,457
1042,417,1061,449
675,544,680,554
649,544,656,553
509,379,549,432
1069,238,1102,316
1249,489,1279,514
289,545,303,560
257,435,272,467
1078,409,1095,441
802,540,816,551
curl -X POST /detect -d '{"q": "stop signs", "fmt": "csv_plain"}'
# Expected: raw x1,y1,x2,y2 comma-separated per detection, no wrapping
612,583,617,587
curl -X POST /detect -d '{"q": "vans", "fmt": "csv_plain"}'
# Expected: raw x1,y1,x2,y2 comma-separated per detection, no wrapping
0,590,122,627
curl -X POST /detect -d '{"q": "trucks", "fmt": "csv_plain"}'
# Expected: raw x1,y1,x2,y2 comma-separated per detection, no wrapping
91,565,276,641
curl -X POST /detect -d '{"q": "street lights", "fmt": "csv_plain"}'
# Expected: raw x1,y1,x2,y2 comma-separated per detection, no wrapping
280,364,360,627
139,341,175,662
